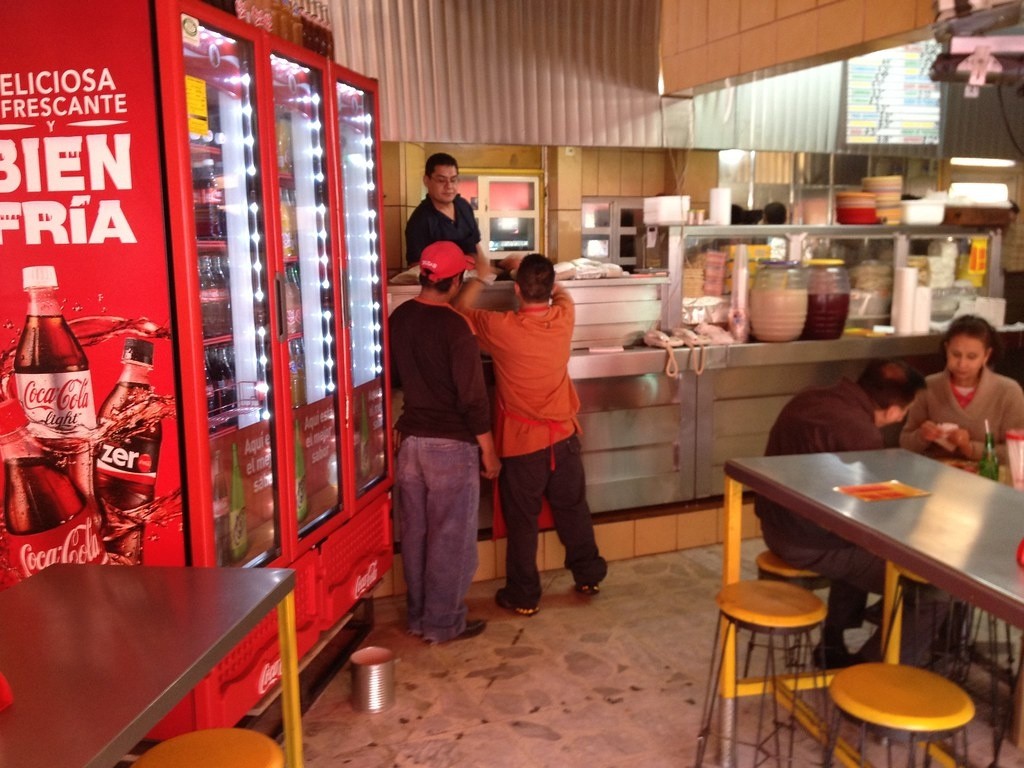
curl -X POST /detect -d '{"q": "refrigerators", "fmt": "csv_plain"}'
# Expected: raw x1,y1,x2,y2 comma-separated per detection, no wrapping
1,0,395,743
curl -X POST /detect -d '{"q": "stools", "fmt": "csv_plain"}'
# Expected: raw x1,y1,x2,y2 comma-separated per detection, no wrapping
127,729,285,768
695,550,1017,768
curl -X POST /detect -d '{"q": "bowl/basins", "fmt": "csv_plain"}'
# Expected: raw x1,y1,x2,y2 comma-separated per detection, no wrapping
836,175,946,226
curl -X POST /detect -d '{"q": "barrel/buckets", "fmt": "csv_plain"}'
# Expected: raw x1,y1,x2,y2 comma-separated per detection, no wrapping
348,647,402,717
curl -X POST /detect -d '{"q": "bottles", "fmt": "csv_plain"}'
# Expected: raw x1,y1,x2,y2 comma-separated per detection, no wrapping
850,259,891,329
750,258,808,342
695,209,705,225
928,237,959,289
0,398,108,576
804,258,851,340
1004,428,1024,494
189,19,303,430
979,433,999,482
12,264,99,504
686,209,695,225
95,338,163,566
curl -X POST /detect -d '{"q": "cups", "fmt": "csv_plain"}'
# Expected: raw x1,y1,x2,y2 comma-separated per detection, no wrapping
729,242,750,342
708,187,732,226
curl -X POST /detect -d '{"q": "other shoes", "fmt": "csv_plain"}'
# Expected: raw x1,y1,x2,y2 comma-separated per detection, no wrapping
496,588,539,616
575,581,599,595
813,643,850,669
426,619,486,645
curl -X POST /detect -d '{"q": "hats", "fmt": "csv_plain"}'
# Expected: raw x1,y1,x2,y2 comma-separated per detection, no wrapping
420,240,475,282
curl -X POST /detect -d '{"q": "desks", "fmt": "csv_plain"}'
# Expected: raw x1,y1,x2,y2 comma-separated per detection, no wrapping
0,564,303,768
719,447,1023,768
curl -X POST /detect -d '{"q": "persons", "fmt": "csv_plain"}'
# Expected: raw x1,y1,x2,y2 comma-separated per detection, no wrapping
754,201,786,244
405,153,487,278
388,241,500,643
753,360,951,668
454,254,607,615
701,204,763,252
900,315,1024,467
